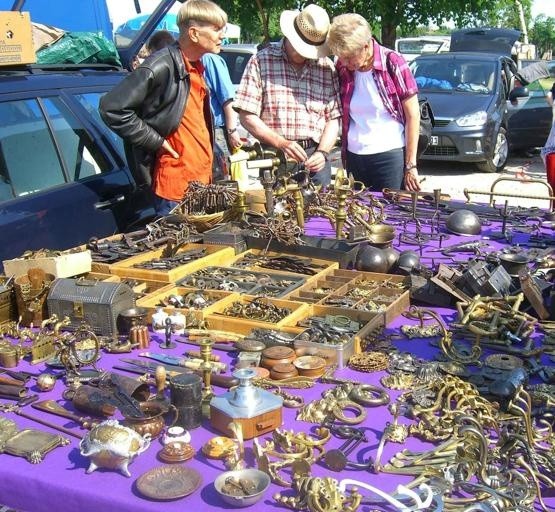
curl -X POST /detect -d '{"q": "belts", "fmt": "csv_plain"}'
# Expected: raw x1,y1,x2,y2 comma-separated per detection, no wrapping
298,138,317,149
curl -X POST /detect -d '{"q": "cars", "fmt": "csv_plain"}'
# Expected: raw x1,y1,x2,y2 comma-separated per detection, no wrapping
0,1,229,284
266,38,286,48
394,27,555,172
216,44,259,134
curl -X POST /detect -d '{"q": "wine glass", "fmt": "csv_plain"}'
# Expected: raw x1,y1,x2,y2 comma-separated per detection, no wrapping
227,365,264,409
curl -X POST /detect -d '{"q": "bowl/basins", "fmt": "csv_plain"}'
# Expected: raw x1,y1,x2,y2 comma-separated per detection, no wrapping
124,401,167,439
214,468,272,508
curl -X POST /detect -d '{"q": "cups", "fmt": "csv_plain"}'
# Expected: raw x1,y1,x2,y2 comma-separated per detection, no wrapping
121,309,151,334
499,253,532,278
368,224,397,250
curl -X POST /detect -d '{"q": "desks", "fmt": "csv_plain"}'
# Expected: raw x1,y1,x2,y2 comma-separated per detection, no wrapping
0,187,554,511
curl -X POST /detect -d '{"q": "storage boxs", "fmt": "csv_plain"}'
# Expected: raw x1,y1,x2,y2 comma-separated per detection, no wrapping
0,9,37,66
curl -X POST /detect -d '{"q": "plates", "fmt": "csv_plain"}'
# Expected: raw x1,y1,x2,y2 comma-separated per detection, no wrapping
135,465,204,501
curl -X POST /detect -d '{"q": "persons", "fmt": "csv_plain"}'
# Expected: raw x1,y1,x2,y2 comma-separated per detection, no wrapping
231,2,343,187
98,0,228,214
147,31,244,175
539,83,555,167
325,13,422,192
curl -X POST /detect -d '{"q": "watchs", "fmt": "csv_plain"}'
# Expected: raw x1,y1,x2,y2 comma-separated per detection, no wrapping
404,161,417,170
226,128,236,135
314,150,330,162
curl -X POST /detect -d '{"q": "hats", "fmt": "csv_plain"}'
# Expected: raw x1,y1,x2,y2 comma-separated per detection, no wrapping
279,3,332,60
